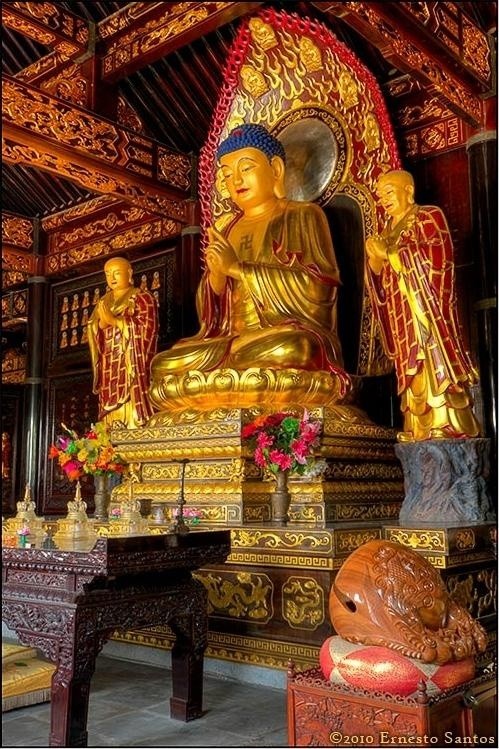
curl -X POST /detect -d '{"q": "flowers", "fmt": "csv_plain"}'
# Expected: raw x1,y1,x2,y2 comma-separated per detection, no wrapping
47,420,129,483
189,510,202,517
183,509,190,517
18,527,30,536
112,508,124,516
241,407,324,477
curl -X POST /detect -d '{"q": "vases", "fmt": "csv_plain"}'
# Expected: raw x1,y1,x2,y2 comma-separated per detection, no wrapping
193,517,199,525
272,471,291,527
21,535,25,548
93,475,111,522
113,515,118,520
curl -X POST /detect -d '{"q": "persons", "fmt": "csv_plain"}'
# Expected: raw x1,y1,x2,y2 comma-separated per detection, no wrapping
143,123,376,429
87,256,160,428
364,170,488,443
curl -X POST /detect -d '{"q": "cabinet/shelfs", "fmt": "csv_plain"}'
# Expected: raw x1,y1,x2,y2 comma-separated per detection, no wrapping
286,650,498,749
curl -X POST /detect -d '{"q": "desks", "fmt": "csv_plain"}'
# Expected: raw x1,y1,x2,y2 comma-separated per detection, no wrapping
0,530,232,746
